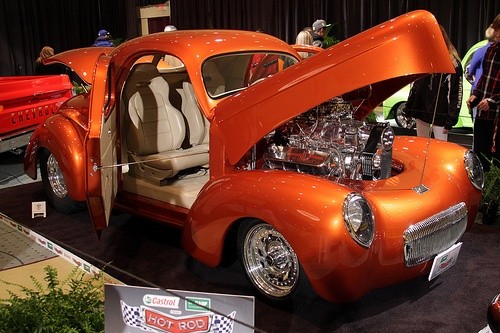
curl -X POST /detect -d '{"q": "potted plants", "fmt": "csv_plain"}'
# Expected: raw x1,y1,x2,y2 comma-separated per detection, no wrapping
478,163,500,226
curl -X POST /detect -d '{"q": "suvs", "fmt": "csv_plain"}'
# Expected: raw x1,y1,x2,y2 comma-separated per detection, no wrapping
25,10,485,310
381,41,490,135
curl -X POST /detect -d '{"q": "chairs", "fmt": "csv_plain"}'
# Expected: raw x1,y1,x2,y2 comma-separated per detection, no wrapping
121,61,226,178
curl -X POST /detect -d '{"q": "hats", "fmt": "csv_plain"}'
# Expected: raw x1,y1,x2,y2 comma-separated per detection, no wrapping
312,19,332,30
98,30,107,36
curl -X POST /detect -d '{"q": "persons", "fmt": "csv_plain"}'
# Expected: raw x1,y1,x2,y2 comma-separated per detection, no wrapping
405,24,463,142
36,46,55,76
466,15,500,231
295,31,314,59
311,20,331,49
91,30,114,47
304,27,313,35
466,23,494,153
